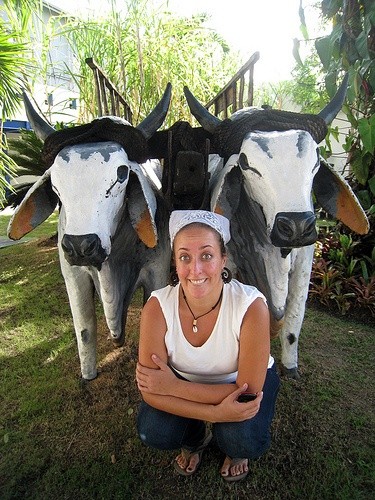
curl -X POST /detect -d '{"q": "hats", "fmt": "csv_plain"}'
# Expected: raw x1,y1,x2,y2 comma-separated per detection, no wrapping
165,209,234,250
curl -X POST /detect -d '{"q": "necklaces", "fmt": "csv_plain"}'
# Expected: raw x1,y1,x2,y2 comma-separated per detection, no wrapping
180,282,223,333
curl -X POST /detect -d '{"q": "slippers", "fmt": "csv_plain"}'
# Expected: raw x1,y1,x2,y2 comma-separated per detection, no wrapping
173,429,214,476
219,453,251,481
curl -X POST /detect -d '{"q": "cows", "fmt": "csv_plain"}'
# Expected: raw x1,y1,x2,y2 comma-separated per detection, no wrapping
7,82,172,381
175,64,369,377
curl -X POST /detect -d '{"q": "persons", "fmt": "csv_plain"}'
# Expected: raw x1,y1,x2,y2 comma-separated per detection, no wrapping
135,209,279,482
